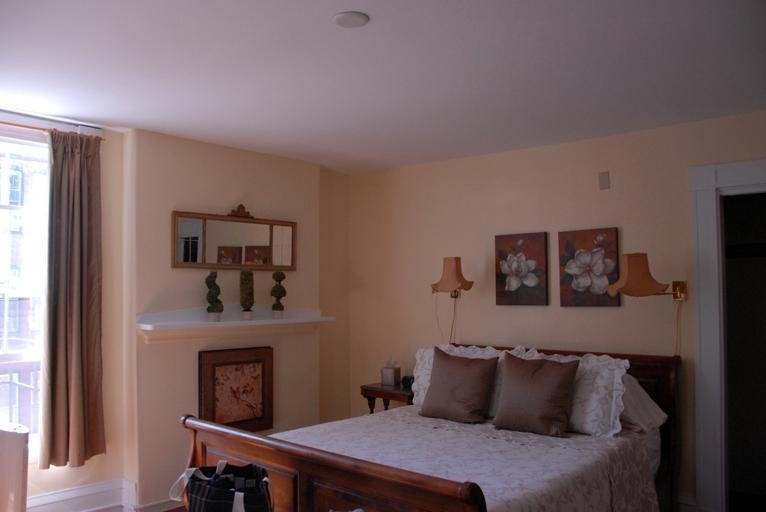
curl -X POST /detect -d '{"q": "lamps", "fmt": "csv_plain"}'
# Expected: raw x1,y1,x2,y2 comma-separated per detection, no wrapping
432,255,473,344
606,252,688,302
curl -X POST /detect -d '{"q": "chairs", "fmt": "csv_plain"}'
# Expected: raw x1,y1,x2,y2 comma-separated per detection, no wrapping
0,427,32,512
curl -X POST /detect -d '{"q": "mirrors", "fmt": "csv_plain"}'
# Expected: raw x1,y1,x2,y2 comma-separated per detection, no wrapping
169,204,297,272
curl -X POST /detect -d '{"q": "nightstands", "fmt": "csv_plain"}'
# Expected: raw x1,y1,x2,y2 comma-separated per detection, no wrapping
360,380,413,414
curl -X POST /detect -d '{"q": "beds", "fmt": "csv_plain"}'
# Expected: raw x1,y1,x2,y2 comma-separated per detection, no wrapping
179,342,684,512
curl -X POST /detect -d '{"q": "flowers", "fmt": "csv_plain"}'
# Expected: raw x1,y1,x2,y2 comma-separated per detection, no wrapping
495,237,546,304
216,245,241,265
244,245,272,266
560,230,619,305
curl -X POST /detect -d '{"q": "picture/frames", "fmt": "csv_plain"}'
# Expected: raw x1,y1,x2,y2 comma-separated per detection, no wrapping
196,345,275,434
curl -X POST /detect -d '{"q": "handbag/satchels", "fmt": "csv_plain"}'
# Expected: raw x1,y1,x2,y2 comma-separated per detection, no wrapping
169,460,273,512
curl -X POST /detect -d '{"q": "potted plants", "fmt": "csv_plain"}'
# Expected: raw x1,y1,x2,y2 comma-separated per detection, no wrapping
270,271,286,320
239,269,254,320
203,271,223,322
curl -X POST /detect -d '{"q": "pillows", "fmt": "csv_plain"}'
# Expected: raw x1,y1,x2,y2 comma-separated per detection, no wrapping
411,344,509,421
416,345,502,424
489,351,582,439
486,344,631,440
